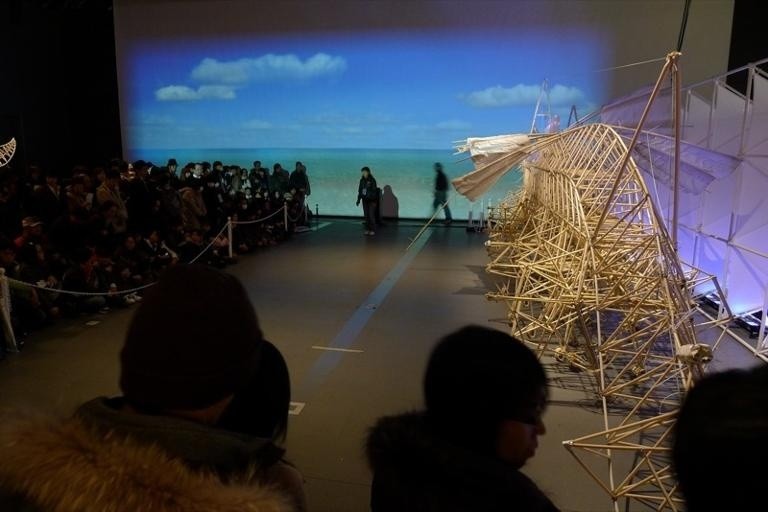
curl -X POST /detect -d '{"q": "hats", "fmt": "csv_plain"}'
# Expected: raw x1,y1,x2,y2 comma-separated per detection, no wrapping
22,217,42,227
167,159,178,166
119,269,261,411
423,326,548,428
133,160,151,170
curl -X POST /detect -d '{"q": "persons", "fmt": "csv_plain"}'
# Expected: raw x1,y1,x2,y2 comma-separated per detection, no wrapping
429,163,453,224
356,166,379,237
670,365,768,511
0,264,292,511
217,336,310,510
365,325,558,512
1,158,310,357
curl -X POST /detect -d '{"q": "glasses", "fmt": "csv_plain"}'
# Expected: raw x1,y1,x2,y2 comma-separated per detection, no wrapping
514,395,546,424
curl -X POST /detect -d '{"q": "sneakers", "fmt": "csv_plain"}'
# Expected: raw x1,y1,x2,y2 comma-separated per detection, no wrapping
364,230,374,235
125,293,141,303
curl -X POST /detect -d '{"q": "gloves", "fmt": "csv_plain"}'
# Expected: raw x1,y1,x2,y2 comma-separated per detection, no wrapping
356,200,360,206
